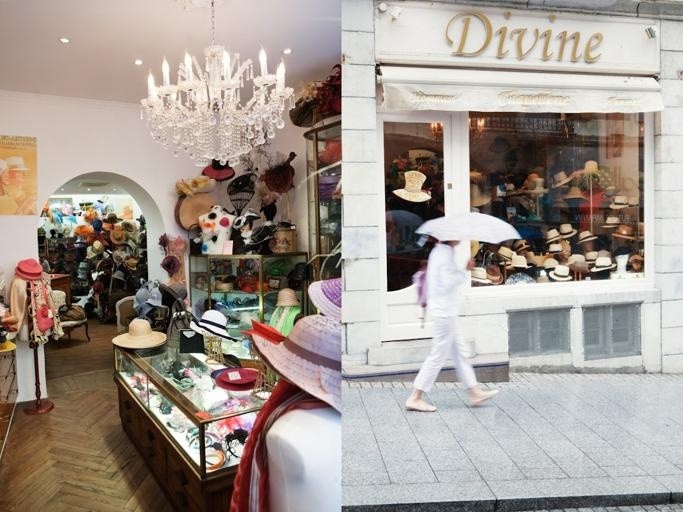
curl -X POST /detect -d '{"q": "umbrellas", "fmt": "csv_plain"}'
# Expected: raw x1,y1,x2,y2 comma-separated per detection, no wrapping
423,212,521,271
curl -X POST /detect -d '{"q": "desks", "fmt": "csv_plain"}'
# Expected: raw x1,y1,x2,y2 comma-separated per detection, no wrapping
50,272,71,306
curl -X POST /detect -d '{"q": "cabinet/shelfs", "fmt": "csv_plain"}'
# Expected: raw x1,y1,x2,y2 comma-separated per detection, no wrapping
0,335,18,458
302,114,341,316
186,253,308,371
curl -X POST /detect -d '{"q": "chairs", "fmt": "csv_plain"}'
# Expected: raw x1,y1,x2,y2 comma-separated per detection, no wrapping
114,295,160,361
49,286,90,344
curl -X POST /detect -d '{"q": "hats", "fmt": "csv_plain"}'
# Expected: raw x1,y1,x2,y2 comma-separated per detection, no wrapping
392,170,433,203
77,212,138,298
158,283,188,303
470,160,642,286
190,158,236,193
0,327,16,352
15,259,43,280
249,277,342,417
190,309,238,344
111,318,168,350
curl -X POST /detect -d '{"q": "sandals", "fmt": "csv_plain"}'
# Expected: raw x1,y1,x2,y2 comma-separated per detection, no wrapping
405,400,438,412
467,389,499,407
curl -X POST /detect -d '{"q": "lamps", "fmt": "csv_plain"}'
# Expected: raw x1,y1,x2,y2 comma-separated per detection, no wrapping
138,1,295,169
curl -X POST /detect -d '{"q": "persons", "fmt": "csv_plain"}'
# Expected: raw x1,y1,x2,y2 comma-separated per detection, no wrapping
7,258,62,343
403,238,497,412
265,398,341,512
0,157,31,214
272,308,303,335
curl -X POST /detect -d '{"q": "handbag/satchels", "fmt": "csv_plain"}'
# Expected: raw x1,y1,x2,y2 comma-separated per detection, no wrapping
58,304,86,321
411,271,428,307
167,311,199,348
36,305,54,332
